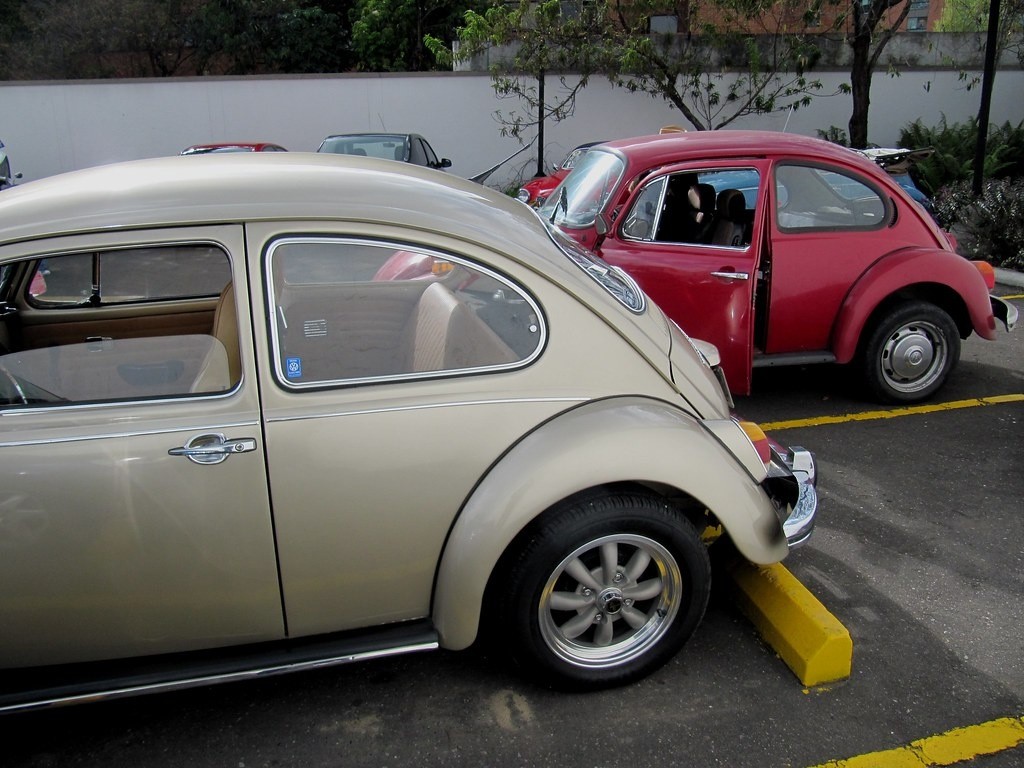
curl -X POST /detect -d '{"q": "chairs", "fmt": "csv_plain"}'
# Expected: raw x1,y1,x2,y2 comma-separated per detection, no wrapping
211,283,243,390
674,183,716,246
707,189,746,246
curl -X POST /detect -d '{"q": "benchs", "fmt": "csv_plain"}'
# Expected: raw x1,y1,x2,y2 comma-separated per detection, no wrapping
403,284,520,369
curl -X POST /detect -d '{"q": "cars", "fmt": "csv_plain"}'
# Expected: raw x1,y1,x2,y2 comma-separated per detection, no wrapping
0,148,822,719
698,142,937,219
177,142,289,156
370,130,1021,406
518,140,633,216
315,129,454,174
